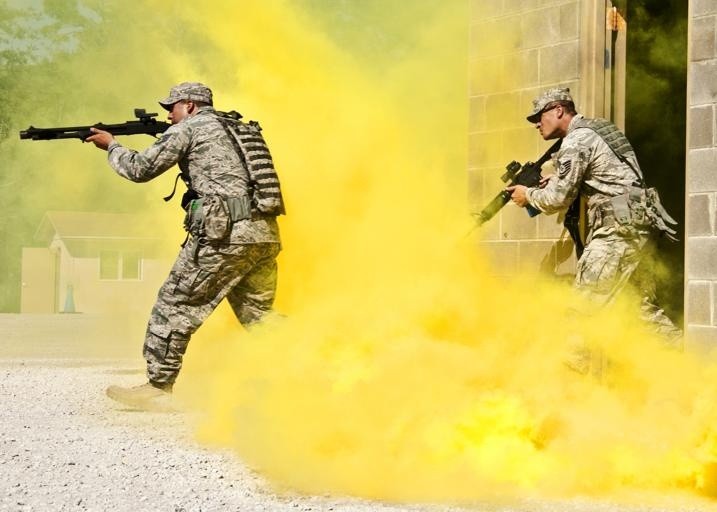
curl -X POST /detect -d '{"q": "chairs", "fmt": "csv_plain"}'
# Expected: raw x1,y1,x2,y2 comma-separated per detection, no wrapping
106,383,173,411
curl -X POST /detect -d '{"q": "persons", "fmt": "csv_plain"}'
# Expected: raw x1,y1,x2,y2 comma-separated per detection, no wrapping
501,85,683,378
81,78,283,416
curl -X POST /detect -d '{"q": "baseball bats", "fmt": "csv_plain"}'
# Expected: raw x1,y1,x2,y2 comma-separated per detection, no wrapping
20,109,172,141
473,140,562,225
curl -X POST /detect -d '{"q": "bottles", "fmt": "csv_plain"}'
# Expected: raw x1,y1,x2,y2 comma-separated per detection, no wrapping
158,83,212,112
527,88,573,123
